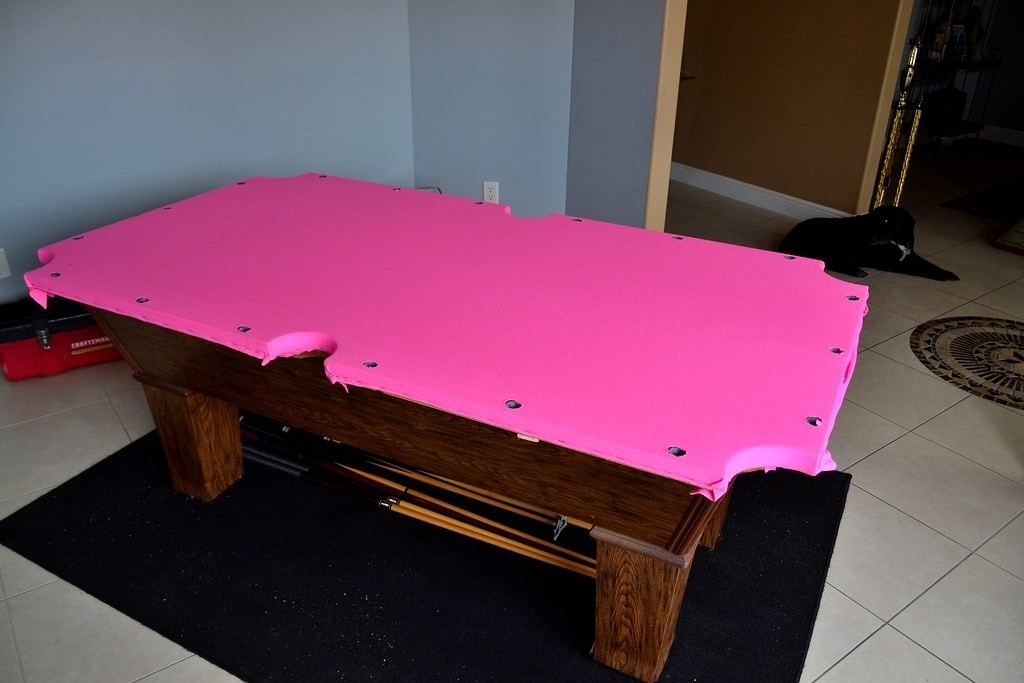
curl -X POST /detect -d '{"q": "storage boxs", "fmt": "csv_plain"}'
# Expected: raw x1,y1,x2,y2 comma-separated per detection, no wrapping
0,296,119,381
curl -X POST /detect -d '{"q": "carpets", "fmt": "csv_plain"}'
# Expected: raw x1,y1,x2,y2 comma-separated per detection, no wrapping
909,315,1024,411
0,409,853,683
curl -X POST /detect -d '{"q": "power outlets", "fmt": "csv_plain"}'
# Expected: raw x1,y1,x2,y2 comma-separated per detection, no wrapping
482,180,498,203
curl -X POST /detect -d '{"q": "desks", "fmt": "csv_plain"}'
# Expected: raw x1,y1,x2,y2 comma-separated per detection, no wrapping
25,172,870,683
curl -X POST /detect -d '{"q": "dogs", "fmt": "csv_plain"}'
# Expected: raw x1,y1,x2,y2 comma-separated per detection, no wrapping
778,205,960,282
